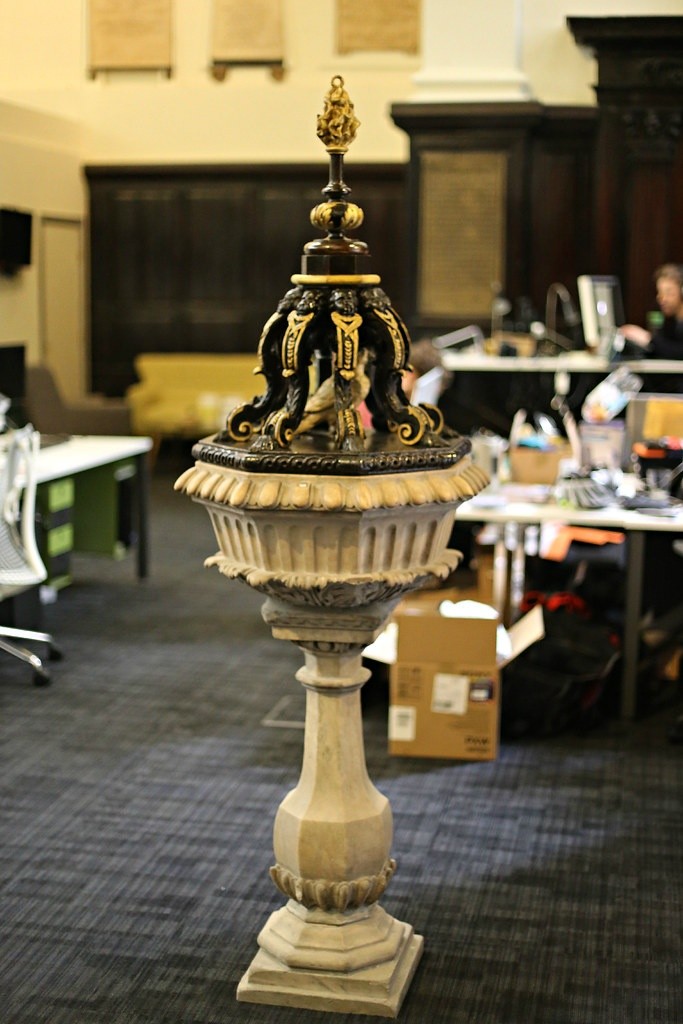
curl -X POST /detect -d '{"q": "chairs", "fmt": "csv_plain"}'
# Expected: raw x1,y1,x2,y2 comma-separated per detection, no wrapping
0,424,63,687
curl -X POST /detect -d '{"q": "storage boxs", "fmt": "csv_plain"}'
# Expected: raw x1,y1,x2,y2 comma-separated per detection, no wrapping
511,447,572,484
361,588,545,762
624,393,683,442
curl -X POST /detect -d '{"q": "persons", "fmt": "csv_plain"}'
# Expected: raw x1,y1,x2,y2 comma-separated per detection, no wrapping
620,264,683,393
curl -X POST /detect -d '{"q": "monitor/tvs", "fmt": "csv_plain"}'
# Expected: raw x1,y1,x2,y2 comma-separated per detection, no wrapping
578,274,619,346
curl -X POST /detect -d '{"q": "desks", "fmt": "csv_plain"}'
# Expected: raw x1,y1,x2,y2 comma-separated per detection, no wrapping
454,475,683,721
0,430,154,631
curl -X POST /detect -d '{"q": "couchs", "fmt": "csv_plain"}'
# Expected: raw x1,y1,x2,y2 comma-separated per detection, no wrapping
128,355,267,448
